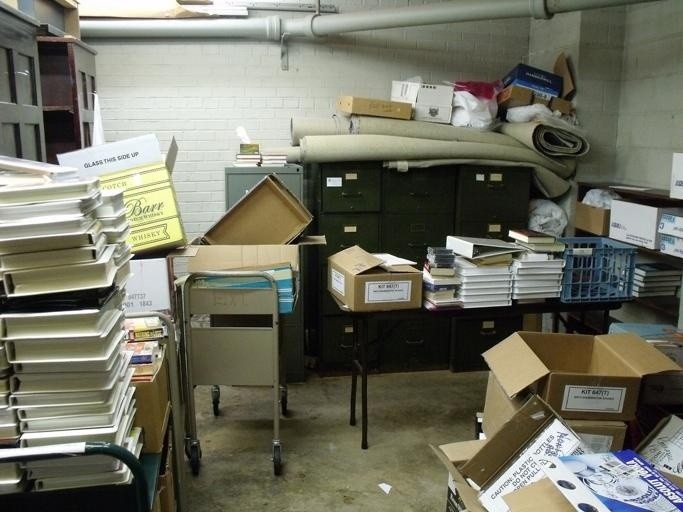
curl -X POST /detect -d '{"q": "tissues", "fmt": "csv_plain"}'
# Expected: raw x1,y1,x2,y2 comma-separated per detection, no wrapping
236,126,260,154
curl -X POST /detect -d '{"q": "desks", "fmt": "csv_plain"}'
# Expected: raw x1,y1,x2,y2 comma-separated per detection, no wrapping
330,292,623,449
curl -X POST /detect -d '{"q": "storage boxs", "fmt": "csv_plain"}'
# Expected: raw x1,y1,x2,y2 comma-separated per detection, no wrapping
429,438,492,512
480,371,628,457
503,446,683,511
131,341,172,453
659,234,682,259
326,243,425,313
391,80,455,124
481,328,683,421
156,423,178,512
458,392,593,512
153,490,162,512
185,234,326,295
574,201,611,237
55,132,186,256
551,97,572,115
659,213,683,237
609,198,683,251
498,85,533,107
501,63,564,97
337,96,413,121
634,412,682,491
123,257,172,311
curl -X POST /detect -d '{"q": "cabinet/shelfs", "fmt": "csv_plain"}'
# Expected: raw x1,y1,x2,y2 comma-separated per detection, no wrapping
224,168,309,378
307,160,531,378
0,2,57,160
39,33,97,163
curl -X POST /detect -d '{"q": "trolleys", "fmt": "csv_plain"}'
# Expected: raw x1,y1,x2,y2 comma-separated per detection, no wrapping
178,269,290,479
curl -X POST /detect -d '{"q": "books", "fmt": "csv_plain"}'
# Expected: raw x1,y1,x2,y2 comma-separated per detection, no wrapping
609,252,681,297
0,156,167,492
371,252,421,273
424,230,567,311
234,153,288,168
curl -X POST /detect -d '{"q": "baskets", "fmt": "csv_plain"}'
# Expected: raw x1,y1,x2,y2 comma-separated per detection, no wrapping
563,237,638,302
